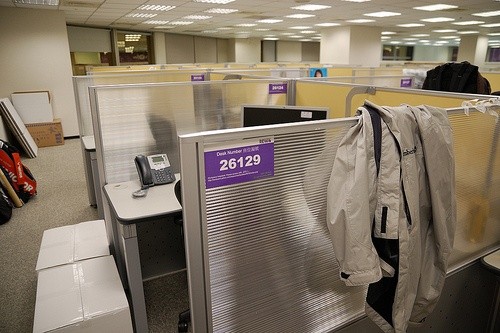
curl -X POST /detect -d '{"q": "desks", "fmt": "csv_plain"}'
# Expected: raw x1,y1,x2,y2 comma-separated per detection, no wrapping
82,134,103,218
102,172,183,333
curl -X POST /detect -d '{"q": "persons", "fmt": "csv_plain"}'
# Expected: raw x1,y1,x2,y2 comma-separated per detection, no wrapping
314,69,323,77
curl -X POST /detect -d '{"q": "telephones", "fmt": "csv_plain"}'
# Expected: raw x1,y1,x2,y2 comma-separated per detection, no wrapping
134,153,175,186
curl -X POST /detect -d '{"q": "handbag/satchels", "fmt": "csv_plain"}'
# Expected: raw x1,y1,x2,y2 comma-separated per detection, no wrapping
402,61,491,95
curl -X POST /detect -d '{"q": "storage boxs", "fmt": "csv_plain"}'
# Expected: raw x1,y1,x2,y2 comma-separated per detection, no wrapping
71,51,101,65
32,255,134,333
25,117,64,148
35,220,109,271
11,90,53,125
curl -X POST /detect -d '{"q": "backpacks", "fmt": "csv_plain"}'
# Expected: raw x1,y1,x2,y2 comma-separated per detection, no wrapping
0,139,37,202
0,191,12,225
